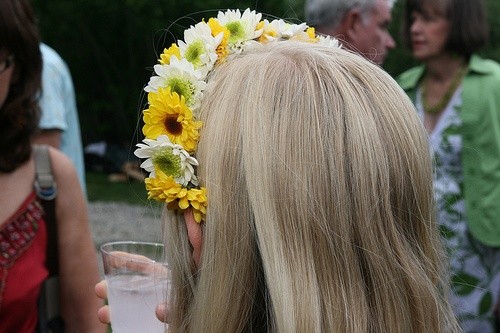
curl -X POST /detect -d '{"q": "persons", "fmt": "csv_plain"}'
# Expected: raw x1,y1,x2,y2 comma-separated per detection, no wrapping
84,6,464,333
304,0,397,67
393,0,500,333
0,1,108,333
30,40,86,196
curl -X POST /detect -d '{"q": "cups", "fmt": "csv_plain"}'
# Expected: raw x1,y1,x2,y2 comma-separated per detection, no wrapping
99,241,171,333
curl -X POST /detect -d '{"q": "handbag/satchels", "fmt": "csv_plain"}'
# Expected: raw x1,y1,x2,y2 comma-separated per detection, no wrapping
32,144,106,333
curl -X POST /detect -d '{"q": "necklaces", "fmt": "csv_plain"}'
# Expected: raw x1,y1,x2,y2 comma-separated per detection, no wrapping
421,60,464,115
